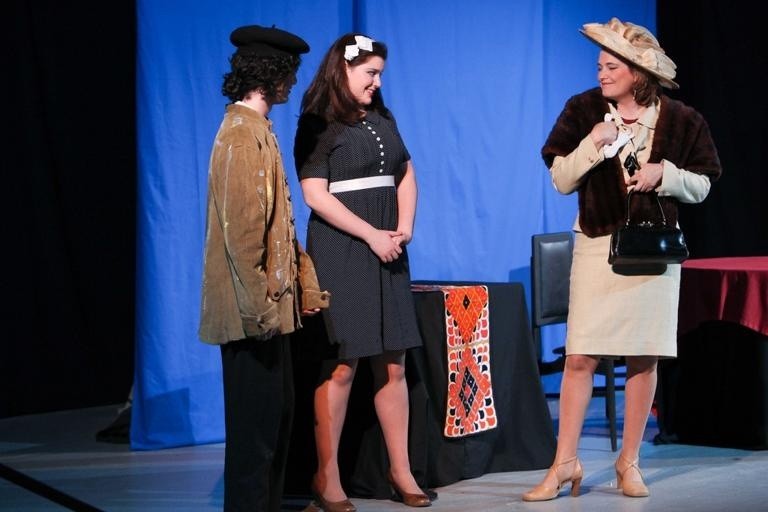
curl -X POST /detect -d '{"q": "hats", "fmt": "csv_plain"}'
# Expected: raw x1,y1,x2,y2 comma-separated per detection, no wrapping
230,24,310,58
578,17,680,90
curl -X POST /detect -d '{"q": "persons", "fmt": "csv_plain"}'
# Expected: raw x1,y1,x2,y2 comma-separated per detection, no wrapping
294,32,431,512
200,24,332,512
521,16,722,500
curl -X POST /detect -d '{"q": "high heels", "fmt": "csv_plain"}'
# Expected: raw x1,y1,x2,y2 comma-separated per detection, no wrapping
522,455,583,502
311,476,357,512
615,454,650,497
386,467,431,506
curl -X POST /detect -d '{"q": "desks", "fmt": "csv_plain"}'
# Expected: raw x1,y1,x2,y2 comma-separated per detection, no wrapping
284,279,558,503
653,256,767,451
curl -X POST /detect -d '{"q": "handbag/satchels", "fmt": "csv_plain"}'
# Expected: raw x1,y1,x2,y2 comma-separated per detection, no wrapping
608,188,690,266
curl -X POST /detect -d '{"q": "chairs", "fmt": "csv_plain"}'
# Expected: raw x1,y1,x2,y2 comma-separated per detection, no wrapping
530,231,628,452
